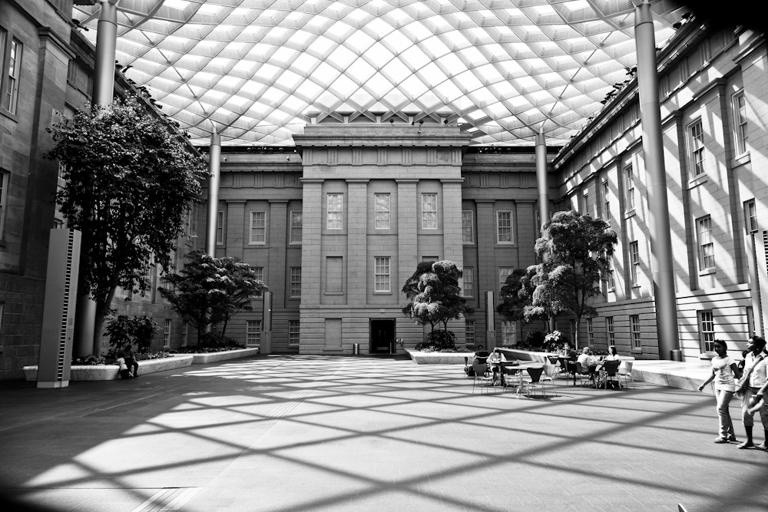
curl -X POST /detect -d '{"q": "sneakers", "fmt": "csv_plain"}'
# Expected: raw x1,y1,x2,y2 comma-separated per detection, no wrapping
713,436,736,443
737,442,768,451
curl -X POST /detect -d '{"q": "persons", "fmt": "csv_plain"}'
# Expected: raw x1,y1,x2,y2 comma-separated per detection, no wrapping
559,345,576,374
486,347,507,387
577,346,597,379
115,353,133,379
698,338,740,445
123,343,139,377
475,343,488,364
736,335,768,450
593,346,620,388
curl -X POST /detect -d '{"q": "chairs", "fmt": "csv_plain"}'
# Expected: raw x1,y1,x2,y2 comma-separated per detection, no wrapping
464,355,635,400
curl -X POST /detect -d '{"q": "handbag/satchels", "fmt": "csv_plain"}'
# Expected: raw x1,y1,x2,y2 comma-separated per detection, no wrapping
735,376,749,393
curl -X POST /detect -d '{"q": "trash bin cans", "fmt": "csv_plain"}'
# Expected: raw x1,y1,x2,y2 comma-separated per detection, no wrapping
353,343,359,355
672,350,681,361
